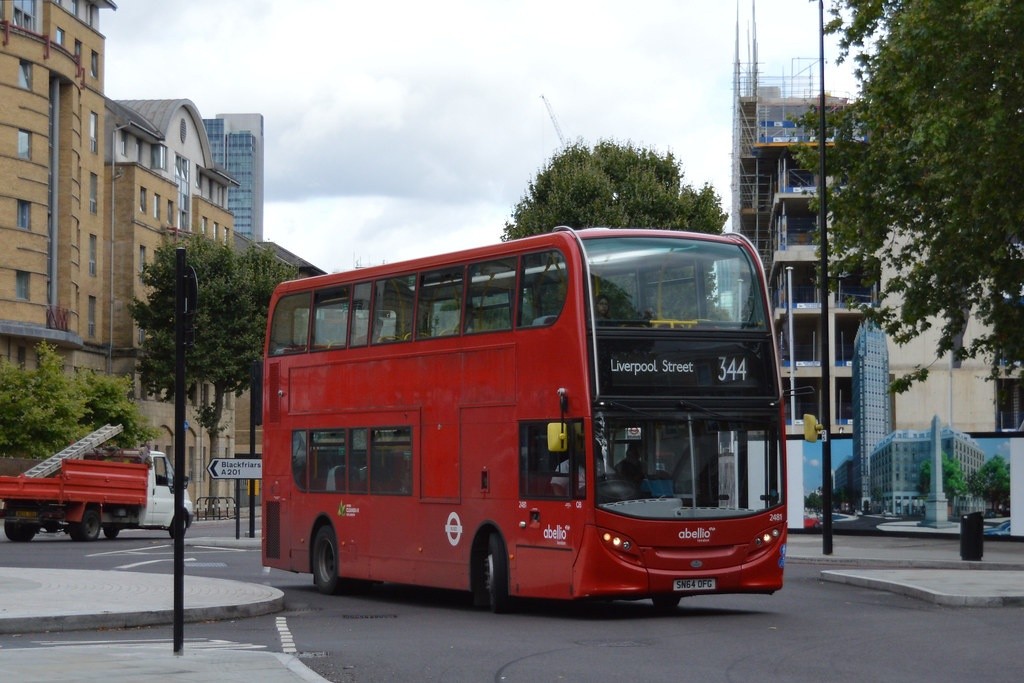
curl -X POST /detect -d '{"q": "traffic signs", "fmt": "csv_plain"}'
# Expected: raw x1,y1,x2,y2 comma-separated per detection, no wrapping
206,458,262,479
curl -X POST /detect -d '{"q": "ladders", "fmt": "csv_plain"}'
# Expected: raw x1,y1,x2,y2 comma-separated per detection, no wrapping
17,423,124,478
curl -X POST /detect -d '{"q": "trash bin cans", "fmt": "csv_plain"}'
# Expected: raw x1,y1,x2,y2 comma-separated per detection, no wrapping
960,511,985,561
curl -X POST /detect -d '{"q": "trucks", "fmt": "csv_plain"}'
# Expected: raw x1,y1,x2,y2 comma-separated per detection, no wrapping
0,424,193,543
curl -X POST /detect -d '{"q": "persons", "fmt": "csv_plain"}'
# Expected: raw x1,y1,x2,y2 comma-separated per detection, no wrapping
614,442,643,481
595,294,610,319
550,443,614,497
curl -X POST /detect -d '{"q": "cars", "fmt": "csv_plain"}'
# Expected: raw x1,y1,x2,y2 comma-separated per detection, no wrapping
804,515,820,528
983,520,1011,535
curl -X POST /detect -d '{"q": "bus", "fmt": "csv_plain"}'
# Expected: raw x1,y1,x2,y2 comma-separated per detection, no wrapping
260,225,824,614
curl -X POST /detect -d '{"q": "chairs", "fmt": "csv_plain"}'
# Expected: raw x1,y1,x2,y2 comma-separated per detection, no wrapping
303,328,455,353
